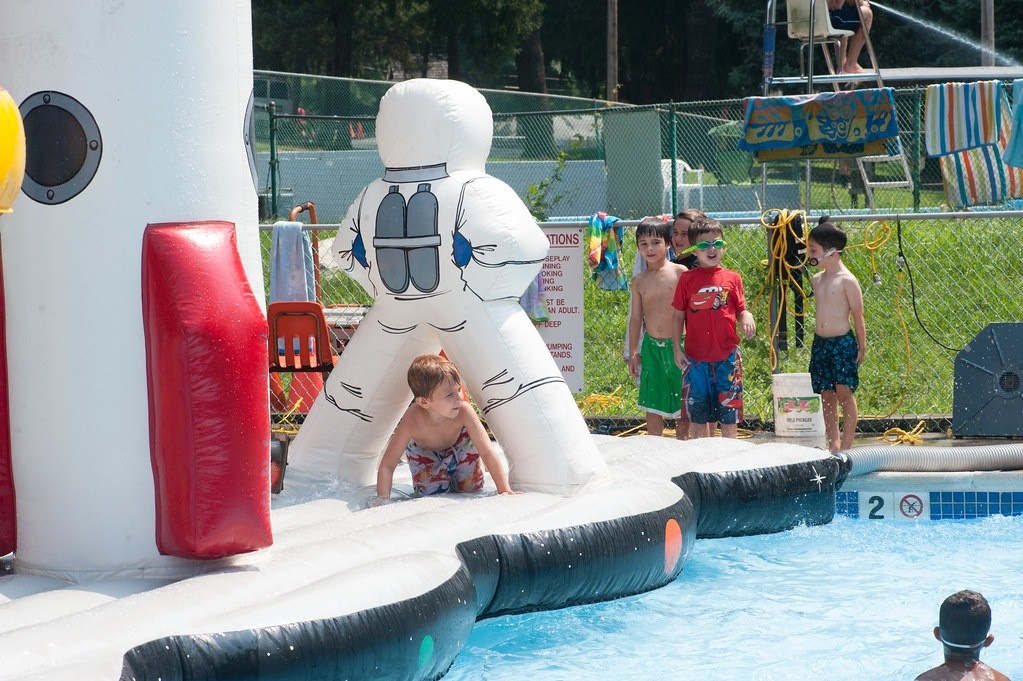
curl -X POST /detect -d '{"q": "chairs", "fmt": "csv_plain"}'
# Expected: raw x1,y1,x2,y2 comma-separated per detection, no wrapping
785,0,854,76
264,301,341,380
660,158,706,217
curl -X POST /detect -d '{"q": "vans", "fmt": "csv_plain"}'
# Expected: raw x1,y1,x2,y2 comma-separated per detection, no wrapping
249,74,295,144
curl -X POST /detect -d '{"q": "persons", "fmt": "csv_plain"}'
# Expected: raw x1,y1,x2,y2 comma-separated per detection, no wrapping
627,218,689,439
672,218,755,439
667,209,717,438
914,590,1012,681
808,223,866,452
377,354,524,507
824,0,872,74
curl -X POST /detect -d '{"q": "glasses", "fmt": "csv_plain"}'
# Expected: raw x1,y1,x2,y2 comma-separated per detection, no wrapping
696,239,723,250
